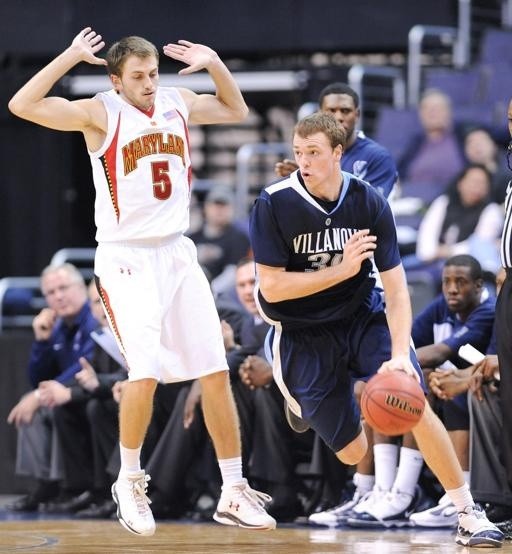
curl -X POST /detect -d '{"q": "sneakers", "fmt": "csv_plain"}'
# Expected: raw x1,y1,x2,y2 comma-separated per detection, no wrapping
308,486,511,547
212,477,277,531
110,470,155,537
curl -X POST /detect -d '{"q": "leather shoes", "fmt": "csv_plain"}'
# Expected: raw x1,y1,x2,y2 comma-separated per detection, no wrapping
8,488,113,517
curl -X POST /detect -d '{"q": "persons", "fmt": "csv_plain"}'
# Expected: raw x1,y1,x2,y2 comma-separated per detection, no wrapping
6,261,349,525
493,96,512,541
248,112,504,547
306,254,503,530
9,26,277,535
275,84,504,264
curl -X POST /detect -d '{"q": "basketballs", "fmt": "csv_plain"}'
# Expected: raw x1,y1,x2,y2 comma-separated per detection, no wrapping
360,371,426,436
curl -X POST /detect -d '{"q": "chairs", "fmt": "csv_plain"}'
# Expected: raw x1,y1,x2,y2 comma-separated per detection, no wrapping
363,0,512,308
